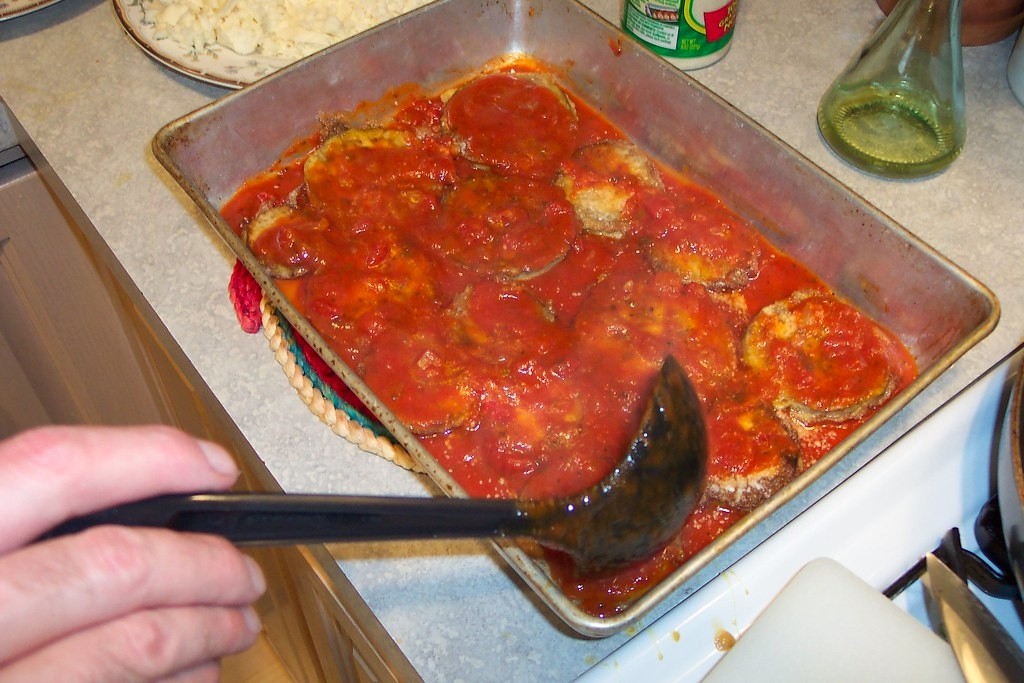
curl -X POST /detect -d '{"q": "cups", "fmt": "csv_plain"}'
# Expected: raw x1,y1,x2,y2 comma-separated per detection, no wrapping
1006,26,1024,107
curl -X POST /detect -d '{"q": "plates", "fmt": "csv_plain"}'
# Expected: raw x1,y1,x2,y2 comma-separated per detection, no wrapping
110,1,443,89
144,1,1000,637
699,557,967,683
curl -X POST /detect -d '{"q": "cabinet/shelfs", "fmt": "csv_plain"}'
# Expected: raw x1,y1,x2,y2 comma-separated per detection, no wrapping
1,145,398,683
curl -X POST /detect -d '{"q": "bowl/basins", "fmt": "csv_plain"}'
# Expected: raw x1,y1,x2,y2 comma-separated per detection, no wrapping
877,0,1024,46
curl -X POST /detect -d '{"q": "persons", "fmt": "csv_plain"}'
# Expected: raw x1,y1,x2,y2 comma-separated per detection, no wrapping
1,424,267,683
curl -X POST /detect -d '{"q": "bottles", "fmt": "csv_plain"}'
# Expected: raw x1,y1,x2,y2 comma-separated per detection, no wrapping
623,0,739,70
817,1,967,178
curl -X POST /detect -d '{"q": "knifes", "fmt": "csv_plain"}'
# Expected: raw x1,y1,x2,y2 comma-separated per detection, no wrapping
924,552,1024,683
